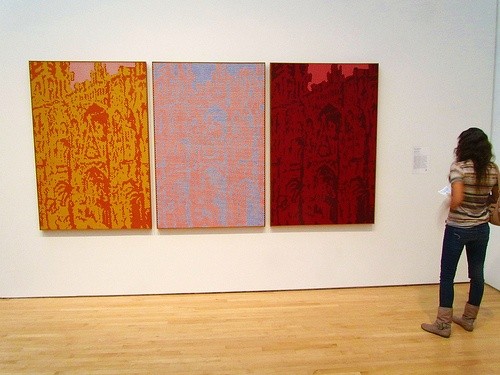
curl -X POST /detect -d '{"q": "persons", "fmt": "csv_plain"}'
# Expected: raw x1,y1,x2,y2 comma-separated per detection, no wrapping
421,128,500,338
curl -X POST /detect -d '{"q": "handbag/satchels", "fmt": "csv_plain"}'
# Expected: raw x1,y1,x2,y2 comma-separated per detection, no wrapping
488,191,500,226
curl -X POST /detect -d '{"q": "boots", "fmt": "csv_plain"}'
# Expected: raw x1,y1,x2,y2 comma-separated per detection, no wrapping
452,302,480,332
421,307,453,338
